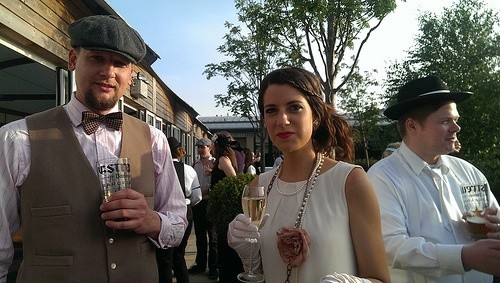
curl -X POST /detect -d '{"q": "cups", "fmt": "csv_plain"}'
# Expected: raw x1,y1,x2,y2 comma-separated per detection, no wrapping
97,158,132,220
460,182,491,240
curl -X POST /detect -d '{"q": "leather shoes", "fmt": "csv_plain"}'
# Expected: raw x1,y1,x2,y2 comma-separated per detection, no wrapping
168,264,220,283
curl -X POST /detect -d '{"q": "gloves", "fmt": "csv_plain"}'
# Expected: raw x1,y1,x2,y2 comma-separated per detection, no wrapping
227,212,269,283
319,272,373,283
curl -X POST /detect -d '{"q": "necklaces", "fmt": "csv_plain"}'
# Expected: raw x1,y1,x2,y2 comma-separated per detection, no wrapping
255,151,327,283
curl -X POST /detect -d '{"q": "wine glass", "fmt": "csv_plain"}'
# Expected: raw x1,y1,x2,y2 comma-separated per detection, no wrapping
237,185,268,283
201,162,209,176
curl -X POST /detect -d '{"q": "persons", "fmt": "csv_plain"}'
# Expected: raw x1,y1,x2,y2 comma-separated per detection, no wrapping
364,76,500,283
226,67,391,283
152,137,203,283
186,136,219,281
381,131,402,161
203,132,284,176
1,13,189,283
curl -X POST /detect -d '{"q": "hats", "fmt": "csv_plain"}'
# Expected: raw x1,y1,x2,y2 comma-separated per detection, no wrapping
166,136,181,152
67,15,147,65
382,75,474,120
195,138,212,146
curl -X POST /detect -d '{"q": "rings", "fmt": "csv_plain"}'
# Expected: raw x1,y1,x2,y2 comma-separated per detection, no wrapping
497,223,500,229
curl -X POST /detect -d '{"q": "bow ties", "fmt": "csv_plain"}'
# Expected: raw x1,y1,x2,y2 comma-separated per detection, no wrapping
81,110,123,135
